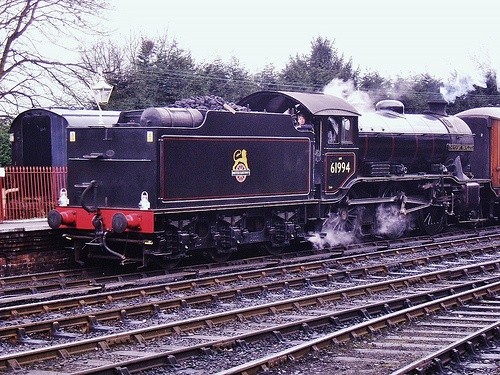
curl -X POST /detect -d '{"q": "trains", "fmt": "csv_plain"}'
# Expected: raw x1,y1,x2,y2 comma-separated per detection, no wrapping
47,89,500,271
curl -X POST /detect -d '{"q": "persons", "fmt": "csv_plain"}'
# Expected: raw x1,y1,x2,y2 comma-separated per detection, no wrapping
294,115,313,132
